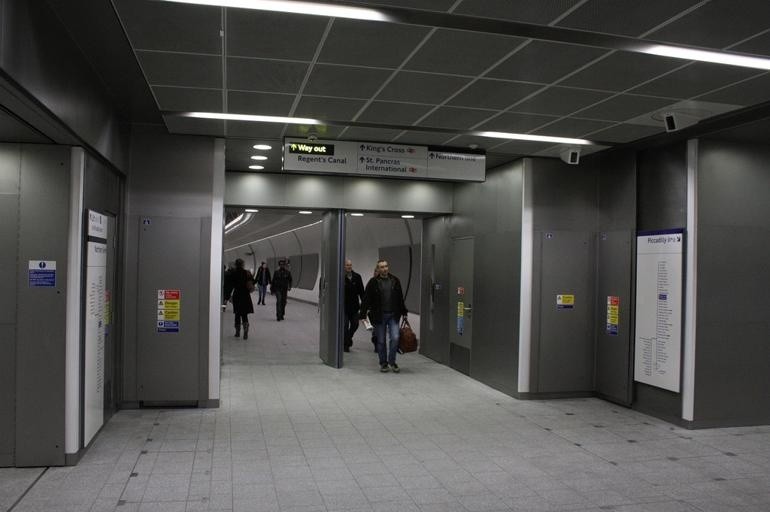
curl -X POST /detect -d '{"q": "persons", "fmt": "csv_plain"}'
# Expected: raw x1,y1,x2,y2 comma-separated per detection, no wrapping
344,258,409,372
223,258,292,340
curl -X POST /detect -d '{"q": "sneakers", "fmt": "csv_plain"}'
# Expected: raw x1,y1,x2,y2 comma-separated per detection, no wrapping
381,362,401,373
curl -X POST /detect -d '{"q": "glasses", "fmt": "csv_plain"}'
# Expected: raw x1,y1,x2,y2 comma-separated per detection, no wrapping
280,263,286,266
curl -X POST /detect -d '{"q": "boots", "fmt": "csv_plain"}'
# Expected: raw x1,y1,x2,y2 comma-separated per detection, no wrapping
243,322,250,340
235,325,241,337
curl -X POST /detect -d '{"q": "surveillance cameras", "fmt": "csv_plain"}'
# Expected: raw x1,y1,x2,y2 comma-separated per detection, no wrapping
662,112,699,133
560,148,582,165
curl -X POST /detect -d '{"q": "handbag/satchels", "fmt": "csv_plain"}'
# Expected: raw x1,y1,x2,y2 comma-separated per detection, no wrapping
246,280,256,293
397,318,418,355
